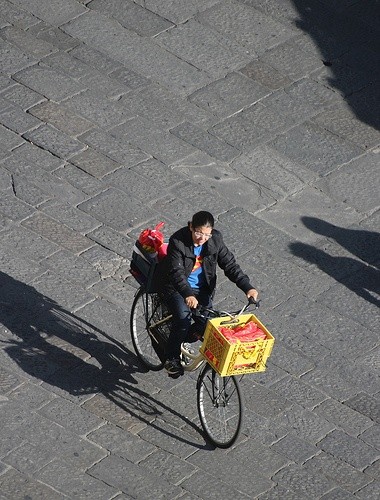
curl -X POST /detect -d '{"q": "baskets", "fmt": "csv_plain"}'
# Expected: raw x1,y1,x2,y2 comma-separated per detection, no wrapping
199,314,275,377
130,240,163,292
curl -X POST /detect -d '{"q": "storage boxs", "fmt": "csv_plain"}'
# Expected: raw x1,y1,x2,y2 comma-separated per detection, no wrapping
130,240,169,293
199,313,275,378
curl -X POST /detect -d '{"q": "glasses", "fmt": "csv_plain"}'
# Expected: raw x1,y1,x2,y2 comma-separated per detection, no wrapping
194,228,212,238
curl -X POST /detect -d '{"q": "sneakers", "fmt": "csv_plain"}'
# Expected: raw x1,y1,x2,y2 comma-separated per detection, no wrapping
162,345,181,374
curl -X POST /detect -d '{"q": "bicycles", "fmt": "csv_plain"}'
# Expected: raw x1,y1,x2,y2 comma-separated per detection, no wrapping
130,240,275,451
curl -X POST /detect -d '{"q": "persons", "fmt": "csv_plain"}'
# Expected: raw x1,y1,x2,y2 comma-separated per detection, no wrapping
154,210,259,375
